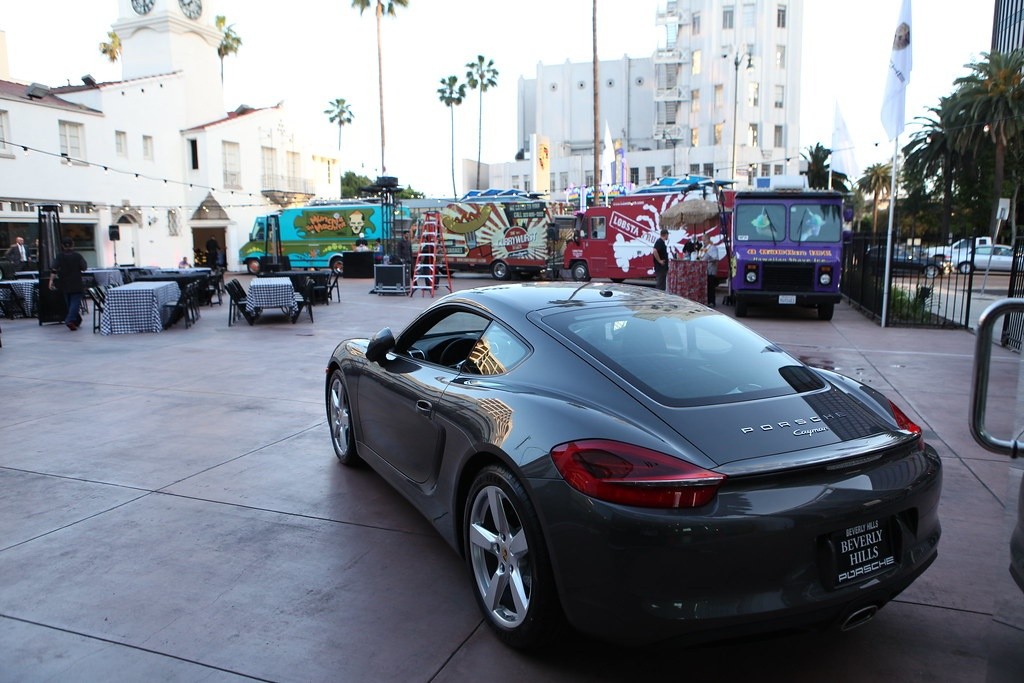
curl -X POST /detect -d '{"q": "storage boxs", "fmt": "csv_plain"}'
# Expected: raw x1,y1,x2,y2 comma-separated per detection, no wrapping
373,264,411,296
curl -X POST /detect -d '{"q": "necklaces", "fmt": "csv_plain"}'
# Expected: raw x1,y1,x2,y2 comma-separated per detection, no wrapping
698,242,713,258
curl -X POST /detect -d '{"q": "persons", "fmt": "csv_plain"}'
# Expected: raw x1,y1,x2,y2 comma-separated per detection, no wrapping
179,234,224,273
653,229,668,292
4,237,40,281
751,215,776,238
48,237,87,331
356,231,439,291
682,234,718,308
801,215,826,242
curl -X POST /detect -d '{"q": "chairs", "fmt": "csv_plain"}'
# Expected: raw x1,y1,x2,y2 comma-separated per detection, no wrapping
0,263,342,334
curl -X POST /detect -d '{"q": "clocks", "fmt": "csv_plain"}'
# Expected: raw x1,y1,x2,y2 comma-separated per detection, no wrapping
131,0,155,15
178,0,202,20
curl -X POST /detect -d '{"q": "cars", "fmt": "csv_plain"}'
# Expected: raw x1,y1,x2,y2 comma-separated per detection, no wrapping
878,236,1024,276
322,278,946,657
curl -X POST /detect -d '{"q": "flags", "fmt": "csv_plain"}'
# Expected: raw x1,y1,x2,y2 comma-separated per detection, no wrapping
600,124,616,191
880,0,913,142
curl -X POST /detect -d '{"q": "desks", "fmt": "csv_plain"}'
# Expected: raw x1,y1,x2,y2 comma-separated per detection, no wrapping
274,271,334,305
99,282,181,337
0,279,39,318
15,271,39,279
156,268,212,277
667,259,708,307
134,273,207,292
245,277,304,324
81,270,124,287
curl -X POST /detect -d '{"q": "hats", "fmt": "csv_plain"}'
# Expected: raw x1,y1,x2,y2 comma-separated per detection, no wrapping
691,236,698,243
661,229,671,234
62,237,73,247
375,238,381,243
359,233,364,238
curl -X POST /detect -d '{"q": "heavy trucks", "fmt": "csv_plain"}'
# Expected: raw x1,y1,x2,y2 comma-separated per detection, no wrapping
728,190,844,319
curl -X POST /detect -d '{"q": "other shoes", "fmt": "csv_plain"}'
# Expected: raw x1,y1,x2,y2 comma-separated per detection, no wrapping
66,322,77,331
707,303,714,308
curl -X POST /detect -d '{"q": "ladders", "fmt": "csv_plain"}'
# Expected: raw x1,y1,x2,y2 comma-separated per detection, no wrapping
409,211,453,298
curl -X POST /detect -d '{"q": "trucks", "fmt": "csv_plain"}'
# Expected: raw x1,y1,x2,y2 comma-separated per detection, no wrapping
237,193,395,275
559,198,729,292
394,199,563,279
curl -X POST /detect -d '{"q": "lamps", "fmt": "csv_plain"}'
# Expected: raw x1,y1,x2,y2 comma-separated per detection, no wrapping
26,82,50,100
82,74,96,87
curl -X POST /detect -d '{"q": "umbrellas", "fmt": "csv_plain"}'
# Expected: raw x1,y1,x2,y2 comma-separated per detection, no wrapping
661,199,731,241
